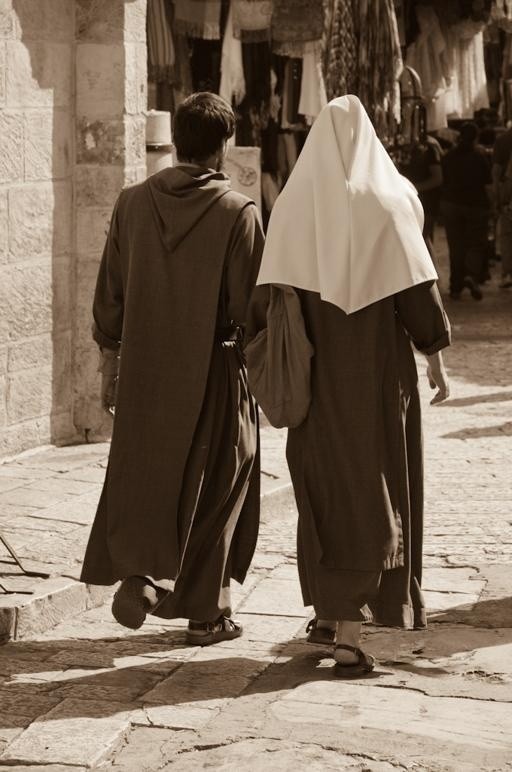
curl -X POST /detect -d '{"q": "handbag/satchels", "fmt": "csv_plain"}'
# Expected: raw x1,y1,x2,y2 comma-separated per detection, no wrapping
242,299,316,430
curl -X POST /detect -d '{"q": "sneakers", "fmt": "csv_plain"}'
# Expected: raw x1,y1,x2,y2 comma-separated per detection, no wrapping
449,274,510,302
110,574,159,629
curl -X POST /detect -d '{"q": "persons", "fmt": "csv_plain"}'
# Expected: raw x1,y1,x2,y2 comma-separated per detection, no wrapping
244,95,450,677
408,107,512,298
80,91,265,644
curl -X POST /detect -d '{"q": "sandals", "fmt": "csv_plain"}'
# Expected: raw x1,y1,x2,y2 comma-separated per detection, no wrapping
185,615,243,645
333,644,375,677
304,616,336,645
